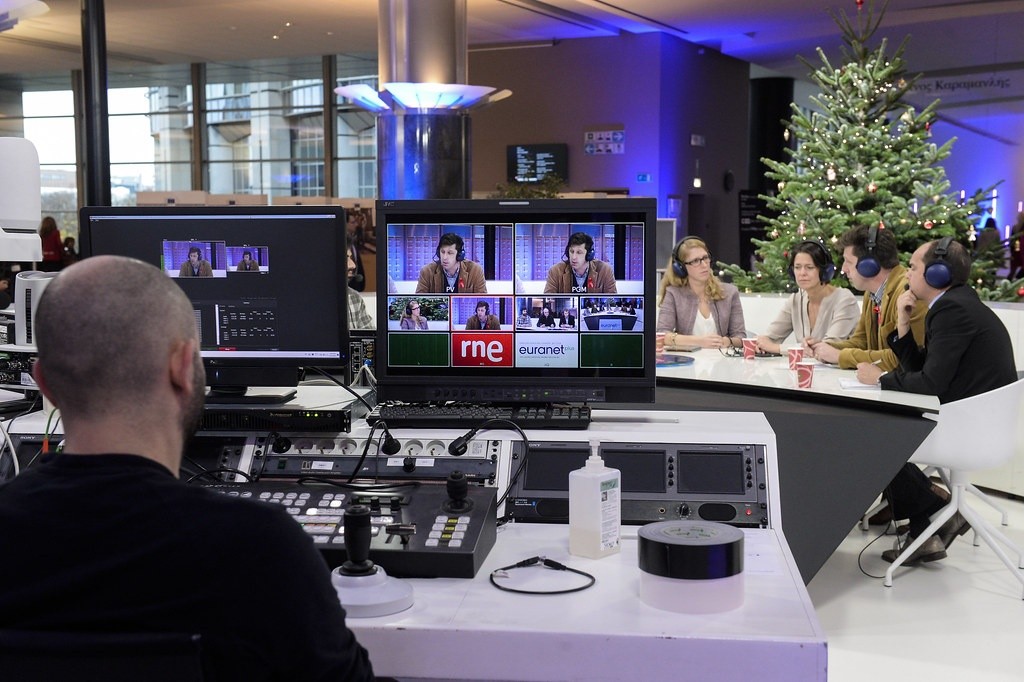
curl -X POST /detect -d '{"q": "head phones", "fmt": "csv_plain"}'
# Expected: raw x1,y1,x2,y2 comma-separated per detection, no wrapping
475,303,490,315
406,300,415,315
188,253,202,260
788,240,836,283
856,221,881,278
671,235,714,279
923,236,955,289
436,239,465,261
565,236,595,262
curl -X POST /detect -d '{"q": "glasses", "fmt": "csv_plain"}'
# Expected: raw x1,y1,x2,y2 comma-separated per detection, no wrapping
684,253,711,267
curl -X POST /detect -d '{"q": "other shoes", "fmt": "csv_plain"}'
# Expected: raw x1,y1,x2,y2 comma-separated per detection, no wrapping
896,485,952,535
880,531,947,566
935,512,970,549
860,502,893,525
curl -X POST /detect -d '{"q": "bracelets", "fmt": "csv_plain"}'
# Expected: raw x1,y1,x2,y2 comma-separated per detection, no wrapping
877,371,888,388
671,332,679,348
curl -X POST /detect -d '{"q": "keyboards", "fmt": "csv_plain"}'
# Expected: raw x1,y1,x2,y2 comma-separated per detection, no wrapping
366,400,592,432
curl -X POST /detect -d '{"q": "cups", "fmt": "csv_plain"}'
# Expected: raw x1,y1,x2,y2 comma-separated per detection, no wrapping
788,350,804,370
795,362,814,389
656,332,665,355
742,338,758,359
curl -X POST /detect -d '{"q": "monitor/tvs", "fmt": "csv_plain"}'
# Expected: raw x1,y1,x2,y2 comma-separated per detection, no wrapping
81,204,348,403
375,197,656,405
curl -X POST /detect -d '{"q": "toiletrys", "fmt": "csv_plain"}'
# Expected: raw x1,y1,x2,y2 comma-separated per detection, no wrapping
568,436,622,560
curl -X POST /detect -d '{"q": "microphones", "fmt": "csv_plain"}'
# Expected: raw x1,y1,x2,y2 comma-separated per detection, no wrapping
713,264,721,276
561,252,570,265
432,253,441,264
904,283,910,290
788,278,799,293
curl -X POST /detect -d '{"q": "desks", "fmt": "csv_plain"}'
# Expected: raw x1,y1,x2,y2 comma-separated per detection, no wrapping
349,407,828,682
657,347,942,588
583,309,638,331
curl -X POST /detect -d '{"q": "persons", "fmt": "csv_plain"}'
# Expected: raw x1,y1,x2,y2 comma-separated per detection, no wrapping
978,212,1024,283
0,254,399,682
581,303,636,321
400,299,429,330
559,308,575,328
544,232,617,293
755,240,861,356
0,217,75,309
466,301,501,330
416,233,487,293
237,251,259,272
803,220,951,526
517,307,532,327
346,211,376,330
656,236,747,350
179,247,213,278
536,308,556,328
857,237,1018,566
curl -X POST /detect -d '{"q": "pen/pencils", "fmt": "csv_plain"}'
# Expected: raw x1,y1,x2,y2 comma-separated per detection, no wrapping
871,359,883,366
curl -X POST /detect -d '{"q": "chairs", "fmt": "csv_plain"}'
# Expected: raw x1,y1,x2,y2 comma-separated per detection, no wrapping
864,371,1024,600
